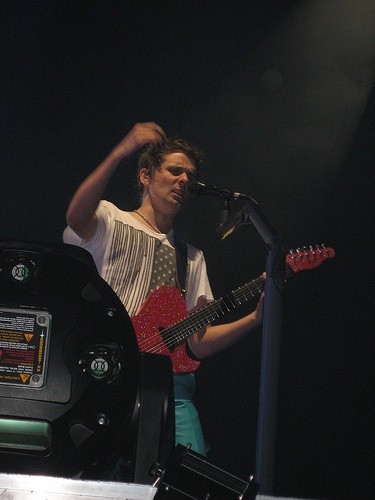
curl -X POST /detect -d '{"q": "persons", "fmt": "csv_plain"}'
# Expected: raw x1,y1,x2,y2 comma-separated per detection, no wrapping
60,122,266,458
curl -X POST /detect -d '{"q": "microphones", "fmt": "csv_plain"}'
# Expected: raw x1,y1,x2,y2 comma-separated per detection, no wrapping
187,181,251,200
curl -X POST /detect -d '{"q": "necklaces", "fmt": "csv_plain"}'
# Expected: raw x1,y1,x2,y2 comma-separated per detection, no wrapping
133,209,162,235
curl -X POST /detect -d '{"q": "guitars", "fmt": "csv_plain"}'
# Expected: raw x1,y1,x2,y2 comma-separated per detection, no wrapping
130,243,335,375
